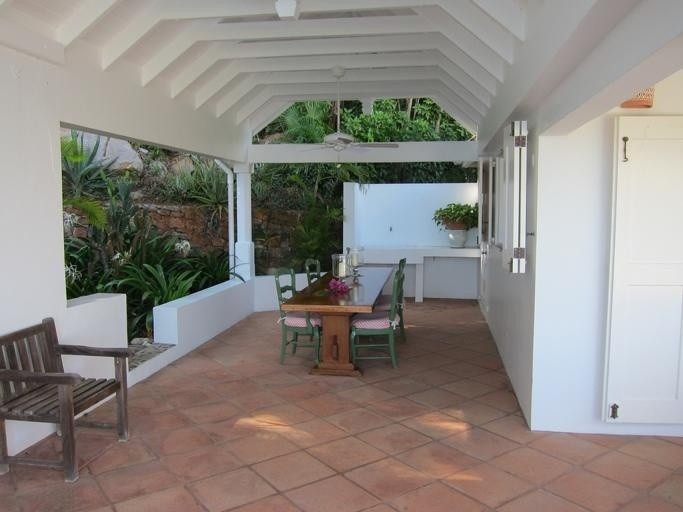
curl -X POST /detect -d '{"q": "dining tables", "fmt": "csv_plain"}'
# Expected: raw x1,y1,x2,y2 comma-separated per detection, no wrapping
431,199,479,248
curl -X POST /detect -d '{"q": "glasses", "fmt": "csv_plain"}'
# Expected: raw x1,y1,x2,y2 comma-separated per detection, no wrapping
275,0,302,21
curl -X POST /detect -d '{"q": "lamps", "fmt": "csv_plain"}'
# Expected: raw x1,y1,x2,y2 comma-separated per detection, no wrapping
274,257,323,367
304,259,322,287
348,256,408,367
0,316,132,484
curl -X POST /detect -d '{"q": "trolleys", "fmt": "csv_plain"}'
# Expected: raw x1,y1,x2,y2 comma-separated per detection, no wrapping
287,64,400,162
